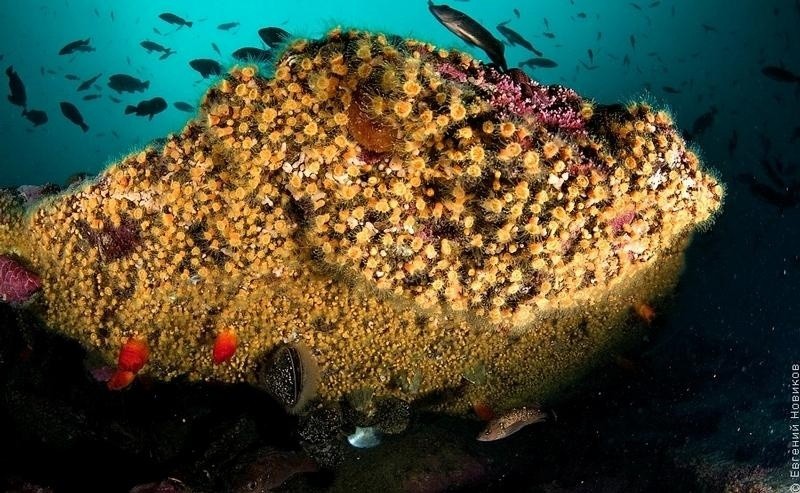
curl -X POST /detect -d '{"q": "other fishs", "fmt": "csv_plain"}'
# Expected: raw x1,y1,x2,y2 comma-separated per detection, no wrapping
0,1,800,213
